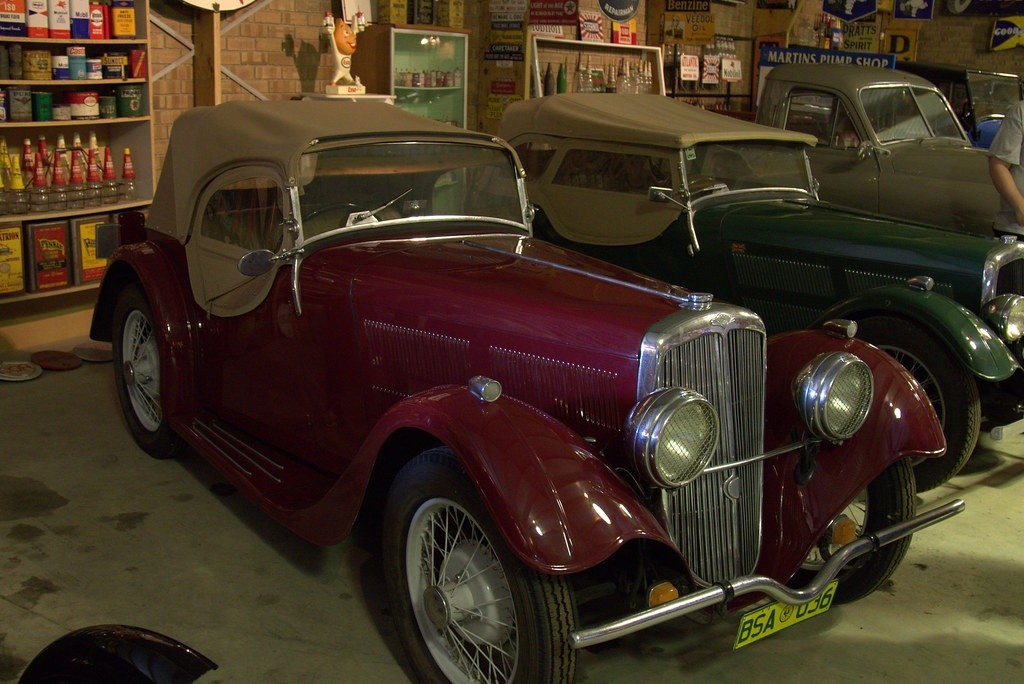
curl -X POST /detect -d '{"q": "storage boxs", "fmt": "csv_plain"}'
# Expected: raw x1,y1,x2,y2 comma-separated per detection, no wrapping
0,207,149,299
378,0,464,28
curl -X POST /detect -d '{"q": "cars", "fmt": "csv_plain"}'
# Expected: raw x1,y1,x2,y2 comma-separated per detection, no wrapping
895,60,1024,149
495,92,1024,494
87,96,966,684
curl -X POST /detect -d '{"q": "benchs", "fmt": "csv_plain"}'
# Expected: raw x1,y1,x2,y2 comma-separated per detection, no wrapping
203,204,400,253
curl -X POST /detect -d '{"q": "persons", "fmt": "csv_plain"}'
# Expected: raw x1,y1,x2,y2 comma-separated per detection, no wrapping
322,15,359,85
986,99,1024,241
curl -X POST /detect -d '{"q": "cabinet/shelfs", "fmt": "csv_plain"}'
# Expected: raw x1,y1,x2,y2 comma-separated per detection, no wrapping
351,23,469,129
0,0,157,305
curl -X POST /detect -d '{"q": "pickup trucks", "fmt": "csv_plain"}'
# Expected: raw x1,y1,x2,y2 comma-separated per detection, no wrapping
704,62,1024,241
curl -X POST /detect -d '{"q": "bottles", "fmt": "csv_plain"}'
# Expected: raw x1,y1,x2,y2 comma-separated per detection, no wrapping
394,66,461,88
814,13,843,50
664,37,737,65
539,51,653,95
1,132,135,214
